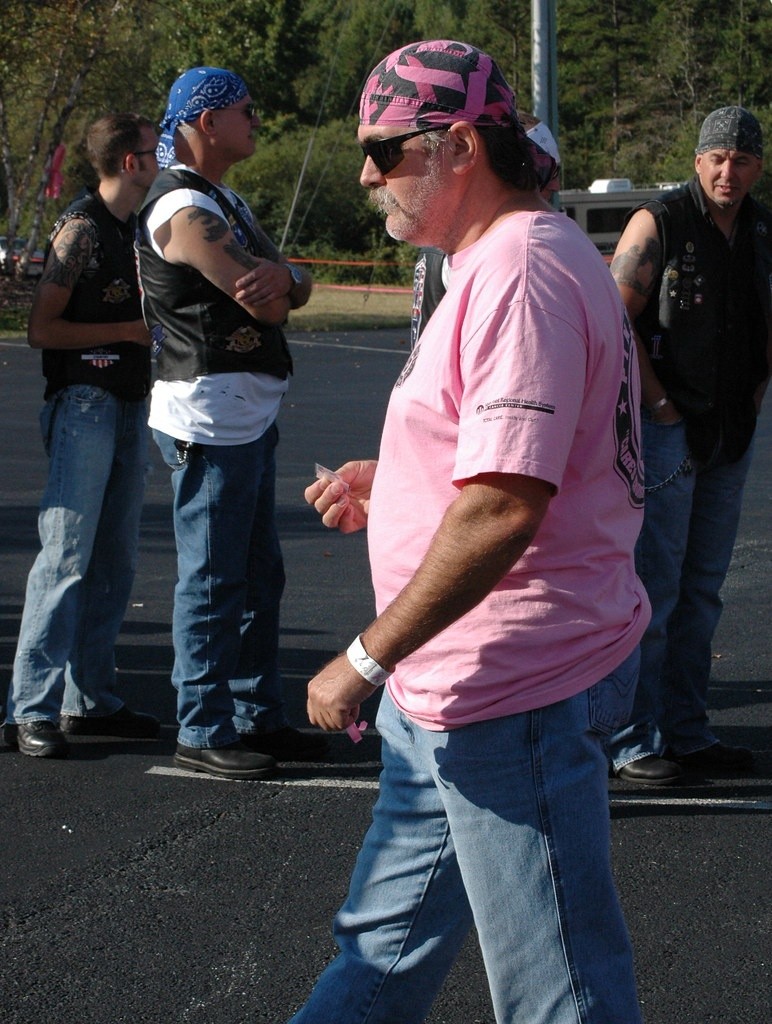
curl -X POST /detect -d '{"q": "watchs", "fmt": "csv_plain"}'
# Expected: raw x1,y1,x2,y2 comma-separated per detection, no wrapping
284,262,303,288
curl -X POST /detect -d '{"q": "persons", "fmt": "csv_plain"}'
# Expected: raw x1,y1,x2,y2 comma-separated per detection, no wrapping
407,109,563,364
132,63,316,782
605,103,771,788
284,32,653,1023
3,110,167,760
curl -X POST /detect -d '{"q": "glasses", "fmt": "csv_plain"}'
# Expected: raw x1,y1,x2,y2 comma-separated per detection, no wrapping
362,126,450,175
215,106,260,118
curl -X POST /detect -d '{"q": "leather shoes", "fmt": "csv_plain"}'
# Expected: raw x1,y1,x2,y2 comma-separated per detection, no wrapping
617,754,681,786
661,742,754,774
173,734,276,779
242,726,329,761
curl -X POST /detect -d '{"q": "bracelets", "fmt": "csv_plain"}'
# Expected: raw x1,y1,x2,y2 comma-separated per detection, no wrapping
346,631,397,690
646,396,668,411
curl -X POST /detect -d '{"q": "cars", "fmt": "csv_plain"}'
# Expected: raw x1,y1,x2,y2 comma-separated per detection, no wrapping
0,236,46,279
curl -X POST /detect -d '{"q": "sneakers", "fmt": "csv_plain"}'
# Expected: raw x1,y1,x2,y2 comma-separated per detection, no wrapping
4,720,67,756
60,705,160,737
